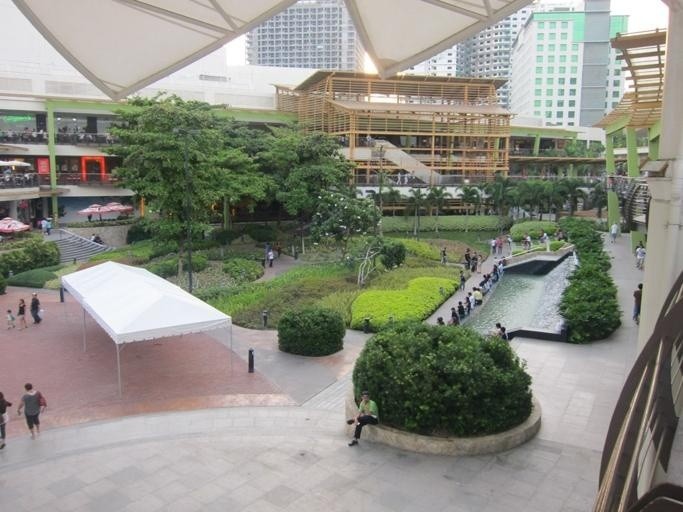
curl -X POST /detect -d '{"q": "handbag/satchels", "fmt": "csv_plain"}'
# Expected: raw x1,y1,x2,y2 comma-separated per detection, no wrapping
39,399,45,406
0,412,10,426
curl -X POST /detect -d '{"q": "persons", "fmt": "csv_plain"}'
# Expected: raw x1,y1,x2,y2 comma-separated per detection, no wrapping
260,240,283,269
0,124,121,144
608,221,621,245
87,213,92,222
631,283,643,326
17,382,47,439
18,213,52,236
5,309,16,330
634,240,645,256
90,233,96,241
0,391,13,451
29,292,42,325
17,298,28,330
634,244,646,270
347,389,379,447
0,167,80,187
93,234,105,244
435,227,568,342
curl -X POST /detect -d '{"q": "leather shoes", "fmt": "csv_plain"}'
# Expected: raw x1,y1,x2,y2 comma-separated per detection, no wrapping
347,419,354,425
348,440,358,446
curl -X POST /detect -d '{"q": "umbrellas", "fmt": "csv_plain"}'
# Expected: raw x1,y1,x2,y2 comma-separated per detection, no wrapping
76,203,113,221
0,160,33,172
103,201,134,220
0,216,31,236
